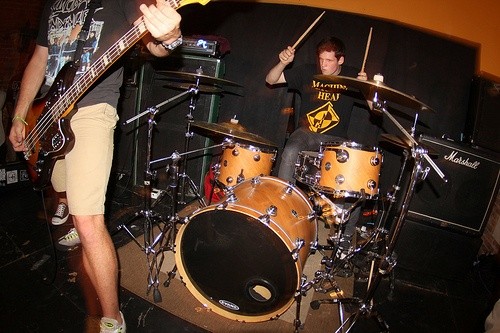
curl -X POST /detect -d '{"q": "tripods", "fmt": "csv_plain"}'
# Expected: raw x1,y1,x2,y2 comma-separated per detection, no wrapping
107,84,419,332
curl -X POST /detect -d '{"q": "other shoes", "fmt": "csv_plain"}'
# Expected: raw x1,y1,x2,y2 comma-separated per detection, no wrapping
322,255,352,278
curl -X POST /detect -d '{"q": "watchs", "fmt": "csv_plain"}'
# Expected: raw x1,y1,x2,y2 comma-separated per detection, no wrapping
161,34,182,51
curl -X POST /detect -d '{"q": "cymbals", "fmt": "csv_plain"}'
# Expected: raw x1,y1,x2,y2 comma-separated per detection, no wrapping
188,119,279,148
167,82,224,93
380,132,440,157
156,69,245,87
313,73,437,114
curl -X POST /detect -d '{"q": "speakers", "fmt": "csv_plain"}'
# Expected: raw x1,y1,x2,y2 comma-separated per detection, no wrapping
112,56,223,196
387,219,483,297
401,134,500,233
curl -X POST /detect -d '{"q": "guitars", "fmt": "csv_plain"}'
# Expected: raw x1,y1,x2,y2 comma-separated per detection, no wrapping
24,0,212,186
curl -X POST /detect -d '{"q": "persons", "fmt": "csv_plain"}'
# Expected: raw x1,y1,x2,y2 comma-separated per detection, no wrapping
9,0,182,333
266,37,373,276
51,192,70,225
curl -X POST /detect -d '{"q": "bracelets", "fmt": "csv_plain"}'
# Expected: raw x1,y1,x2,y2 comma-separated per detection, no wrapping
12,116,28,125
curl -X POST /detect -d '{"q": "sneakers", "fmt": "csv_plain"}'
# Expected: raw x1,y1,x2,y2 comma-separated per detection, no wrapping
57,227,81,246
100,311,126,333
51,202,69,225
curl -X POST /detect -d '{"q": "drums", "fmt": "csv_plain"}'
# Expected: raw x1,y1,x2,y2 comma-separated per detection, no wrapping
176,177,318,323
293,149,323,189
318,143,385,199
210,137,278,190
